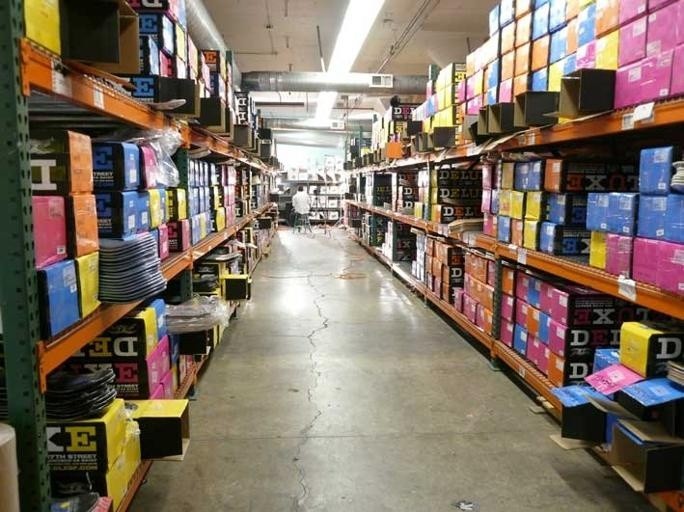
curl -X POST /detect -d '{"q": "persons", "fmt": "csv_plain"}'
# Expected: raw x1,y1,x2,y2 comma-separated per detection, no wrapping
291,185,311,233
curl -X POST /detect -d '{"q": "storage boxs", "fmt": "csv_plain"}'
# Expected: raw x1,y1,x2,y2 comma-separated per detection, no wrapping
464,1,682,512
19,0,285,510
341,60,464,332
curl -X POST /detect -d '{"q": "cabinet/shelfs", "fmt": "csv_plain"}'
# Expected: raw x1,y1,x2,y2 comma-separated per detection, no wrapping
278,181,341,224
1,1,280,510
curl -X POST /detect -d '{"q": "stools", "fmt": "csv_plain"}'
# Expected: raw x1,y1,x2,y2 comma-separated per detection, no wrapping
293,211,311,232
